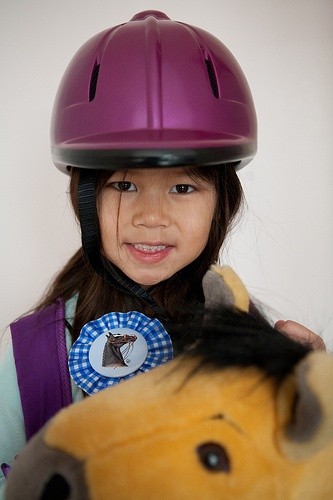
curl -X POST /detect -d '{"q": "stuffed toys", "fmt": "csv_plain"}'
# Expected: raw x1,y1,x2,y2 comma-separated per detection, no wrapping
1,264,333,499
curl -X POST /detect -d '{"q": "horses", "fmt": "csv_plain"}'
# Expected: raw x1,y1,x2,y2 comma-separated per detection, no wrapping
102,332,137,369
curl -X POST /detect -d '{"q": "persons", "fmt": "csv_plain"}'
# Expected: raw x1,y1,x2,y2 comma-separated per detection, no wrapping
1,9,327,499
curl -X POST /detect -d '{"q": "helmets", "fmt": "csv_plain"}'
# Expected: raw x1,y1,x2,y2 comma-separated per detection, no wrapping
51,10,256,174
